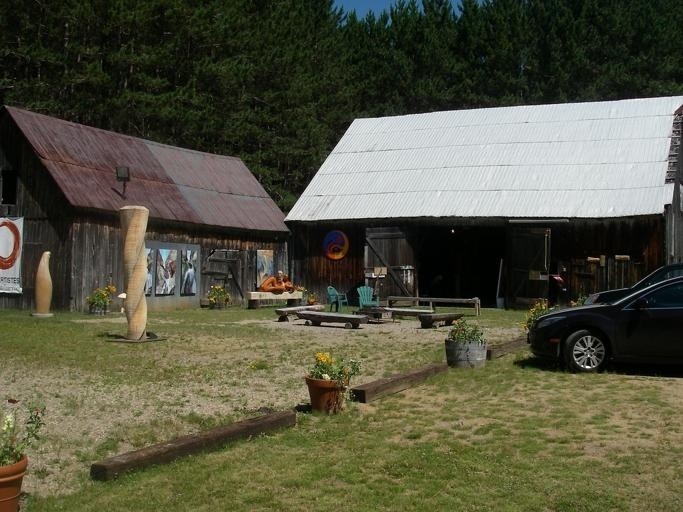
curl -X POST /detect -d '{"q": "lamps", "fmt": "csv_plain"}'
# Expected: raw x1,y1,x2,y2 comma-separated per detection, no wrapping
114,165,130,183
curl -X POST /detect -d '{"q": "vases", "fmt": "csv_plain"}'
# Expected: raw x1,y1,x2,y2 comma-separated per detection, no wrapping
0,453,28,512
445,339,488,370
209,301,227,309
89,304,106,314
305,375,350,416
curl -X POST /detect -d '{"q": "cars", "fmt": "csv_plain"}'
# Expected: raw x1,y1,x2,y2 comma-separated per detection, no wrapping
527,273,682,374
581,263,681,305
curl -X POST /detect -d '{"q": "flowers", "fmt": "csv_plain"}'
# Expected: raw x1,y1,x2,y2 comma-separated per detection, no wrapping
85,284,117,307
308,346,362,382
449,317,486,342
208,284,231,304
0,398,46,467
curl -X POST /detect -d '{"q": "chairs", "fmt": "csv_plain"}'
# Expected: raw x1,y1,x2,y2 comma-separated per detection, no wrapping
357,285,379,309
327,285,349,312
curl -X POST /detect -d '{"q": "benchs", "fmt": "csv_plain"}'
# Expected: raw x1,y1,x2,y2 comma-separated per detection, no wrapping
299,310,368,327
380,306,434,318
389,296,480,315
419,313,463,328
275,305,325,322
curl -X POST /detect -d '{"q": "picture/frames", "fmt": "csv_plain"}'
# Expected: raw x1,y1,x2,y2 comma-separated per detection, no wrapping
180,250,197,295
155,249,177,295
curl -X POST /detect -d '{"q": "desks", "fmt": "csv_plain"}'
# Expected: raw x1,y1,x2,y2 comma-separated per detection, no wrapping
246,290,303,308
